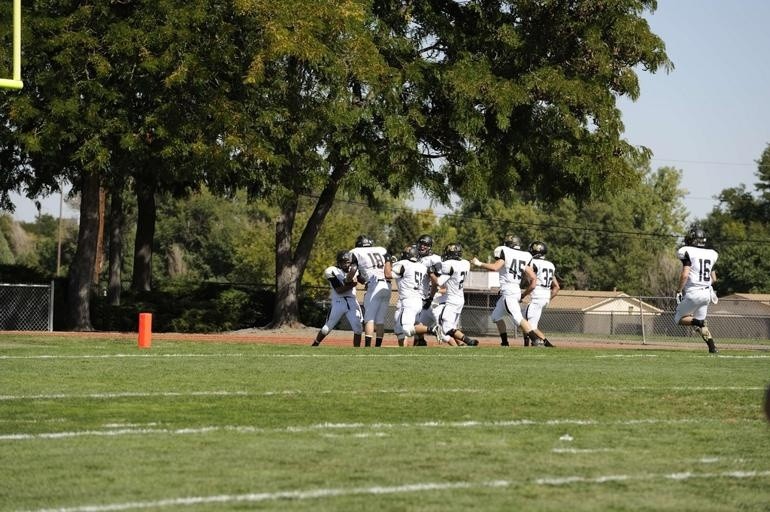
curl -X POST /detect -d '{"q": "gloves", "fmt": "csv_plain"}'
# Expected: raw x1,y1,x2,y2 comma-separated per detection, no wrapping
675,291,682,304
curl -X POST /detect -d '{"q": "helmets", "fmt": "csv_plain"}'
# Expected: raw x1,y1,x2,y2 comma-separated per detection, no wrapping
337,235,548,270
685,227,712,247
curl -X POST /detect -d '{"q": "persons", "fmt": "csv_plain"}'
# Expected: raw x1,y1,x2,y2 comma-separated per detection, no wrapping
472,237,542,347
674,229,719,353
311,233,479,347
523,241,561,349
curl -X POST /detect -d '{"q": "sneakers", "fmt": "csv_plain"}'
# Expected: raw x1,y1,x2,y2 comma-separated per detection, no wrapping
468,340,479,346
710,345,719,354
534,338,543,347
690,317,708,327
